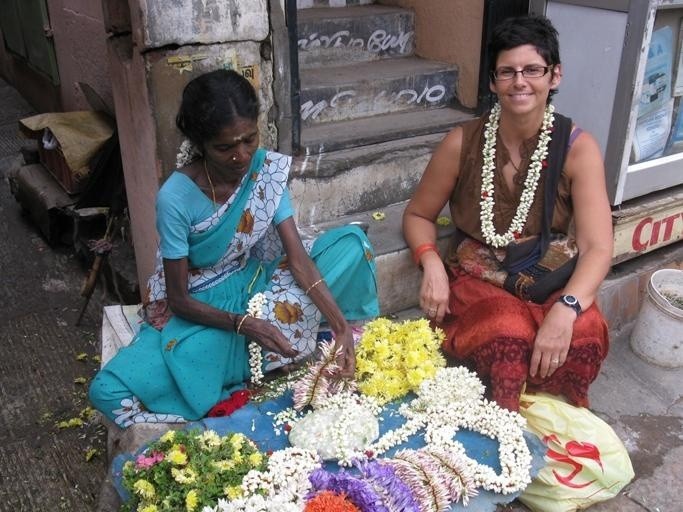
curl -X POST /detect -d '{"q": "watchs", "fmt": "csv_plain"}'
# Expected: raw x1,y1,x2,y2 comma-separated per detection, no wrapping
558,293,580,315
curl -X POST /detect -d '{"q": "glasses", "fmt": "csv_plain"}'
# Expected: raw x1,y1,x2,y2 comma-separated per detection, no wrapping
487,62,554,84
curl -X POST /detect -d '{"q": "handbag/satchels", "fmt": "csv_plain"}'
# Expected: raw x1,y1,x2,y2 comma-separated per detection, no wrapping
452,226,584,301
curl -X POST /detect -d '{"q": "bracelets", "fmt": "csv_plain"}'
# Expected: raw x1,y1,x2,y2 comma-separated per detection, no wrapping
234,314,246,331
412,238,440,263
305,277,325,295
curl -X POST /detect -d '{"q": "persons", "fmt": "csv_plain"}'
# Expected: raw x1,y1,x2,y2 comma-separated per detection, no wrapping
88,69,380,429
402,17,615,411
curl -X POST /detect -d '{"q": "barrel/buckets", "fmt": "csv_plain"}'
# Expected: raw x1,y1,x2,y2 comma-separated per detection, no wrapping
628,269,683,370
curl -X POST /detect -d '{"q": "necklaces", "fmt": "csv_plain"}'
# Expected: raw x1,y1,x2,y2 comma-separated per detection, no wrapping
504,153,527,184
202,158,217,214
480,101,555,246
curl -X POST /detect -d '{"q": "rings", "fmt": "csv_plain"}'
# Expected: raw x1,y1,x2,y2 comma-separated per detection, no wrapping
549,356,559,365
